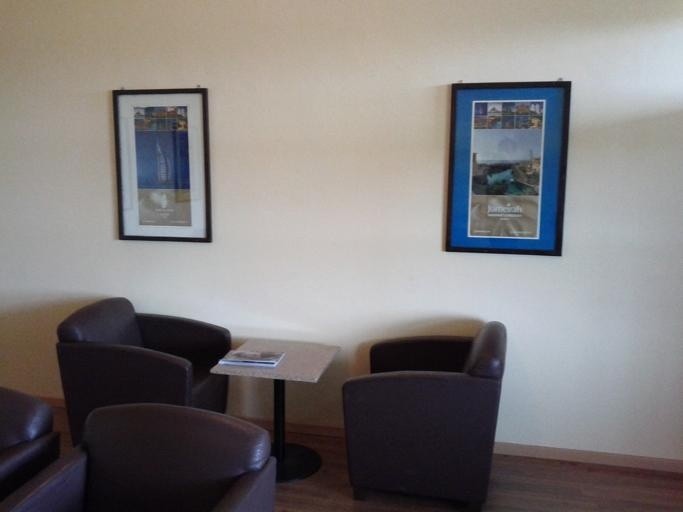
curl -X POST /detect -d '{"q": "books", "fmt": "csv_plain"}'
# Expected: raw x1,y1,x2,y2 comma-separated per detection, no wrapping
216,350,287,368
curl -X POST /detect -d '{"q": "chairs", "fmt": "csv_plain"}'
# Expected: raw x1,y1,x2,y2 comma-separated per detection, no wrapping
0,386,61,502
55,296,232,448
342,321,507,512
0,402,277,512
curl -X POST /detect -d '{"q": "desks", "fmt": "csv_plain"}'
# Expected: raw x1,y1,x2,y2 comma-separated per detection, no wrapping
209,338,341,480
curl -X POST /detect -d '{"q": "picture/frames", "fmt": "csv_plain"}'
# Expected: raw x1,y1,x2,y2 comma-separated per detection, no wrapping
112,88,212,243
445,82,571,256
